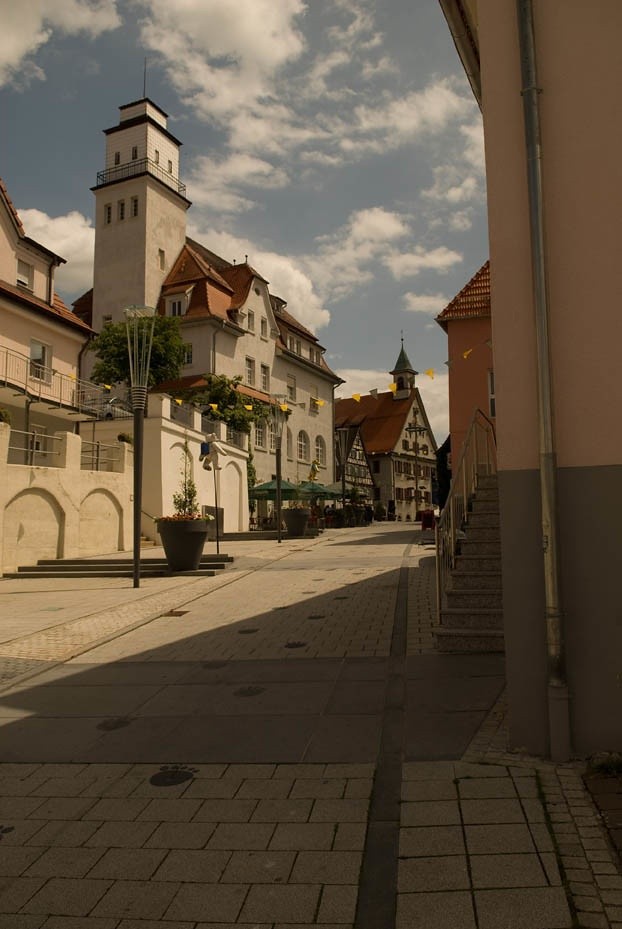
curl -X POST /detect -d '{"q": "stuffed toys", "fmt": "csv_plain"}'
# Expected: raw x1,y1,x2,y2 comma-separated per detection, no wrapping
199,433,227,471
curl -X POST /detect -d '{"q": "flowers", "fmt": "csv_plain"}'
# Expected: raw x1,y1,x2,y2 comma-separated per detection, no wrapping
152,427,215,523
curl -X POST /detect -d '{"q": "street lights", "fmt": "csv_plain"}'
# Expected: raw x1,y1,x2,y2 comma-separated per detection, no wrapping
336,427,349,528
268,391,289,545
121,302,162,589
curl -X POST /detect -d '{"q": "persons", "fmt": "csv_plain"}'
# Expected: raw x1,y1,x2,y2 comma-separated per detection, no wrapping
309,504,373,526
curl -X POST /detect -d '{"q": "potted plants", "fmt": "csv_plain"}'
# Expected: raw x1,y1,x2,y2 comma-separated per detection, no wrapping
282,501,311,537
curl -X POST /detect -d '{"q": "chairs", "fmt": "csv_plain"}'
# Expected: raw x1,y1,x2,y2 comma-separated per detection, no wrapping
249,518,257,530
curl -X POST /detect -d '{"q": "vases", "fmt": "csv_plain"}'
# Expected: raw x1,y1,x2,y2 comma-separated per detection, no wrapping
158,523,209,571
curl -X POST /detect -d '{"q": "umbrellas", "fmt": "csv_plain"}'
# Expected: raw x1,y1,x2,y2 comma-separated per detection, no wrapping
249,480,356,500
